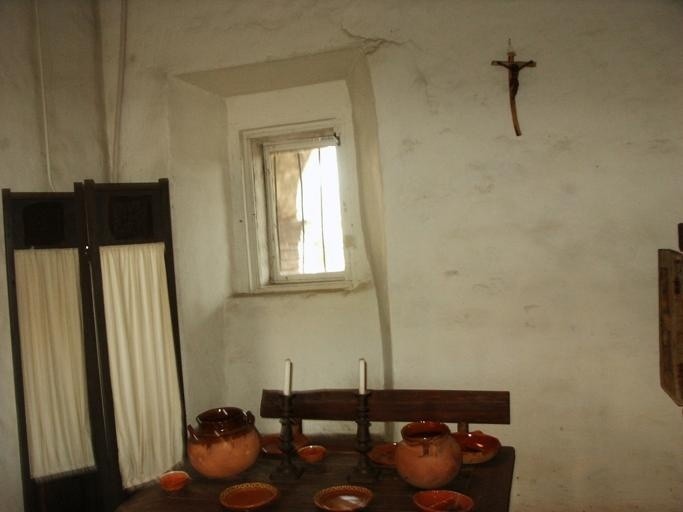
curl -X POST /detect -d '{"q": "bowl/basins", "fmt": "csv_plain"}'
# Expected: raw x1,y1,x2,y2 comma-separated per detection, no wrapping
161,471,192,494
450,432,502,464
296,445,326,461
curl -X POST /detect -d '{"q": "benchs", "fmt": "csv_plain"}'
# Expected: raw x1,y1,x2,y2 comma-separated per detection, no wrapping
260,388,510,439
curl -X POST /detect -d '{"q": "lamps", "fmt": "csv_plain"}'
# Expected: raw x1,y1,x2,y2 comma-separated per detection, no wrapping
489,39,536,136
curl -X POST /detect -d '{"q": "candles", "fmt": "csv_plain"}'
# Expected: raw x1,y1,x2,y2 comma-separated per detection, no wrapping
359,357,365,393
283,360,289,395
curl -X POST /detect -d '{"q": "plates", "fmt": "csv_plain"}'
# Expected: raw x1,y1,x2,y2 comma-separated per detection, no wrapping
263,434,306,457
366,441,397,468
217,481,476,512
